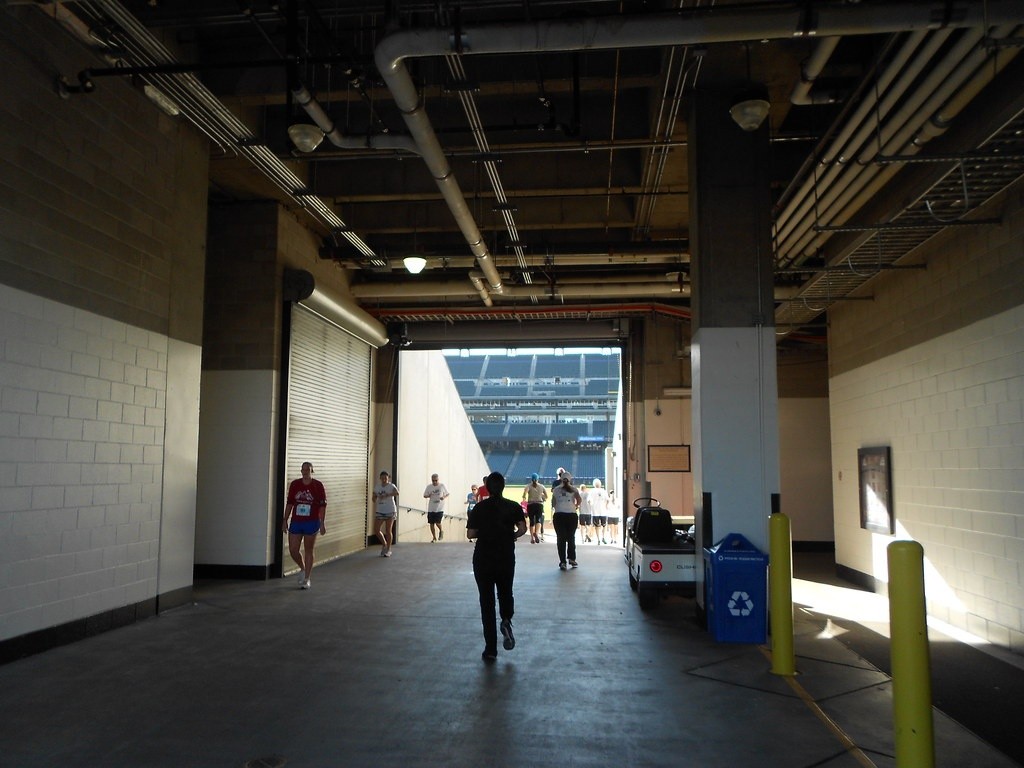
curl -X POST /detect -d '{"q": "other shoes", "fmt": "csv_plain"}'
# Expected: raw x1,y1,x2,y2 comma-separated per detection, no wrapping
533,532,541,544
304,579,311,588
482,651,496,664
431,538,437,543
560,563,567,571
568,559,578,568
610,540,617,544
380,545,388,556
469,538,474,542
499,619,516,651
438,530,444,541
582,533,592,543
384,550,393,557
539,538,545,542
298,569,306,585
531,539,536,544
598,537,607,545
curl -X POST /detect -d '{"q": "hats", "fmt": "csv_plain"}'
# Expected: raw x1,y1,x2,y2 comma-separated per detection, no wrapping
561,471,572,482
531,473,539,480
557,468,565,476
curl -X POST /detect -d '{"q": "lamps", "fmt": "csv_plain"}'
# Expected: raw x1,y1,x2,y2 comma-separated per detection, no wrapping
403,211,428,275
286,124,325,152
726,41,771,133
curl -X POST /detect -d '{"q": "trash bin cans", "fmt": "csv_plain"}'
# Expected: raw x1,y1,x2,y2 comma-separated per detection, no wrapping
704,533,767,644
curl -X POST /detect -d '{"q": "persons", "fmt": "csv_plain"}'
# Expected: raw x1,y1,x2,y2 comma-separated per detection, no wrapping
551,467,573,492
552,471,582,569
476,476,489,503
372,471,399,557
464,484,478,542
540,494,547,543
522,473,548,544
521,497,528,518
423,473,450,543
466,473,528,659
608,490,619,544
587,479,610,545
282,462,327,589
576,484,592,544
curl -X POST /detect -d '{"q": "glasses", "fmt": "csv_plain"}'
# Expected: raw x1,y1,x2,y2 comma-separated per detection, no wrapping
431,479,439,482
472,488,476,490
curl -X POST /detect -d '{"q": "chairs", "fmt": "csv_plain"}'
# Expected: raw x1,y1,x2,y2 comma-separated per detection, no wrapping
634,506,675,545
